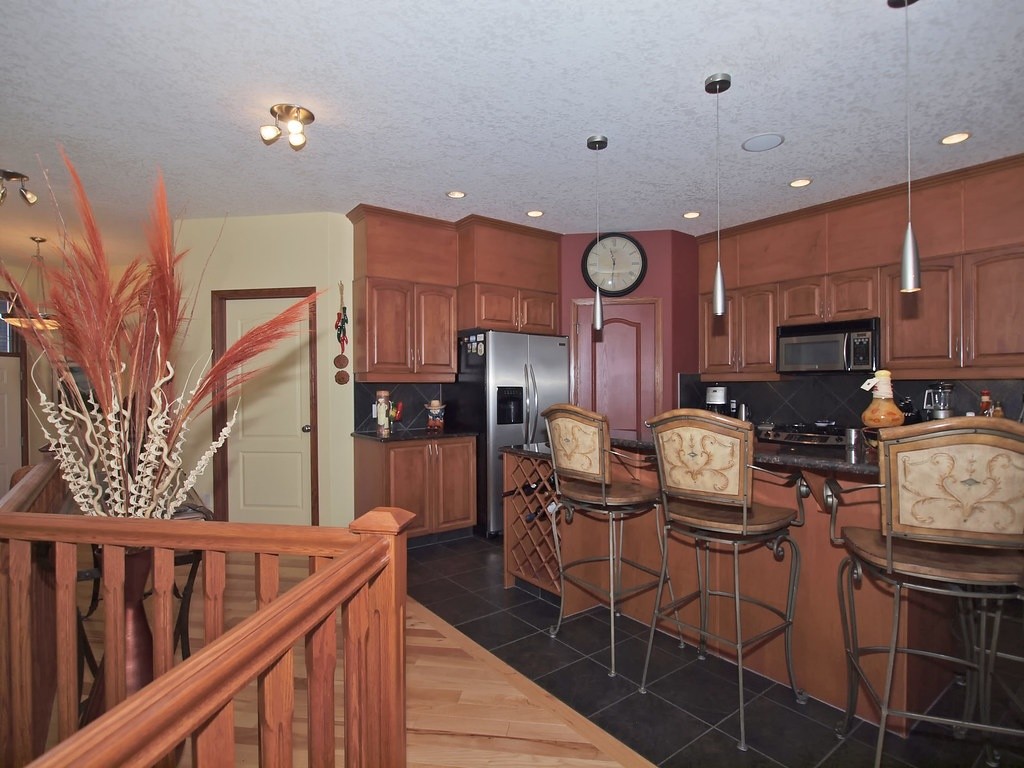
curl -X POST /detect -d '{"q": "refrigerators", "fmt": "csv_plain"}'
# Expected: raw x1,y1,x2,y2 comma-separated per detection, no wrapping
442,329,570,541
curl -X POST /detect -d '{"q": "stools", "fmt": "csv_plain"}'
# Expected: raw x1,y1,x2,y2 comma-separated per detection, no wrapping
540,406,684,679
836,416,1024,768
640,408,810,752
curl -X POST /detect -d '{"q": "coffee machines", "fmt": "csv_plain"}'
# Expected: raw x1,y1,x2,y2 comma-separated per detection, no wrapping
705,385,730,417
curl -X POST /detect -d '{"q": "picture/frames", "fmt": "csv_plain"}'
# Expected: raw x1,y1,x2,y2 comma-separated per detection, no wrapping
50,360,117,419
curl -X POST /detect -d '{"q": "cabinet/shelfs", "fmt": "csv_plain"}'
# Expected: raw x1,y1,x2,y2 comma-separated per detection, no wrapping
354,438,478,551
345,201,562,382
696,153,1024,381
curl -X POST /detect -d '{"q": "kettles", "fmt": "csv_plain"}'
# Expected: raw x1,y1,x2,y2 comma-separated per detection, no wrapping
736,400,752,422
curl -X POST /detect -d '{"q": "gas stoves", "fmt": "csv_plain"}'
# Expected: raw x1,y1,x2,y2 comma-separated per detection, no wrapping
759,424,846,446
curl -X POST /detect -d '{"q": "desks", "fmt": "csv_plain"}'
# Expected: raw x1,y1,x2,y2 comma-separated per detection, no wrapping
46,501,212,742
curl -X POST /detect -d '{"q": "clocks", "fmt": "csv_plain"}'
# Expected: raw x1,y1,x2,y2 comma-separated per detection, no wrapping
581,232,649,297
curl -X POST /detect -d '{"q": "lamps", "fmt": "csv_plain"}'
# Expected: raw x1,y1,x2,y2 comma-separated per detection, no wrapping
259,104,315,150
588,136,608,329
887,0,922,292
0,237,68,328
0,169,37,205
705,72,732,315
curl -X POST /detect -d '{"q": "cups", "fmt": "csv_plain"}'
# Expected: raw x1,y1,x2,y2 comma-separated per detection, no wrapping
845,428,861,447
375,390,391,438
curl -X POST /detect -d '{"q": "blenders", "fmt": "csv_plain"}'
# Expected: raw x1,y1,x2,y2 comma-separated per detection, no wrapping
922,382,955,421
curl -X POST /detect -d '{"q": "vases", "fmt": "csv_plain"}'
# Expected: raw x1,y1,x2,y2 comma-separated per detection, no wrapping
81,549,186,768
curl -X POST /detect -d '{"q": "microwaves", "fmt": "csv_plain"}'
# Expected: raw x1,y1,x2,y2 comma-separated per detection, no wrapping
776,316,881,373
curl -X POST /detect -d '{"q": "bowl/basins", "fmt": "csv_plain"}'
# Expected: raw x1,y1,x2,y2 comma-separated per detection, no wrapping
861,426,889,450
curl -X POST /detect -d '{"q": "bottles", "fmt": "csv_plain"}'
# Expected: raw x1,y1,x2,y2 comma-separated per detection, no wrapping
861,370,905,428
502,475,563,521
979,391,1004,417
